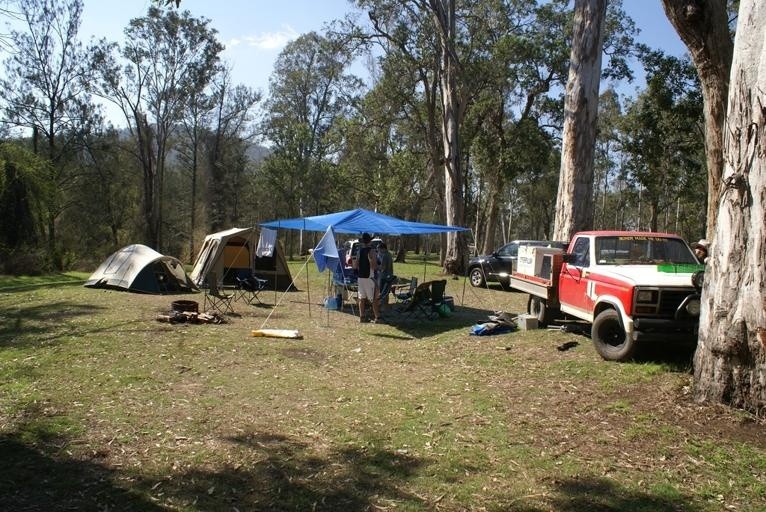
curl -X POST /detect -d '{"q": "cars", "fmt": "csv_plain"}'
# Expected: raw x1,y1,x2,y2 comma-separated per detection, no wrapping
465,240,568,290
343,239,384,269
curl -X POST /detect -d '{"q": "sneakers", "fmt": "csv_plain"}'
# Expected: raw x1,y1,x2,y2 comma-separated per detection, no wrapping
359,316,371,323
374,318,389,325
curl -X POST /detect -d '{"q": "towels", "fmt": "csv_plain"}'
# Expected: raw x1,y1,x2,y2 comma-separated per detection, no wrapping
256,228,278,258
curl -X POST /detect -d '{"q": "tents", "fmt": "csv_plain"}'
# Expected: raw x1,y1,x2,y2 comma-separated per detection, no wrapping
190,227,297,292
84,243,200,295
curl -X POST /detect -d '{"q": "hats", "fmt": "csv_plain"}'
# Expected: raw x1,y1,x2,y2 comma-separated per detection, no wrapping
358,233,374,243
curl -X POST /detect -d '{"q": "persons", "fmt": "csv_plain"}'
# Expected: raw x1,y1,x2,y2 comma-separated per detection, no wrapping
355,233,382,324
376,243,393,313
691,238,708,264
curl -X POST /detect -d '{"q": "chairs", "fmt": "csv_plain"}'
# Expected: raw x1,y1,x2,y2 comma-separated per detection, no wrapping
237,271,270,305
203,271,235,316
392,276,448,321
583,243,647,265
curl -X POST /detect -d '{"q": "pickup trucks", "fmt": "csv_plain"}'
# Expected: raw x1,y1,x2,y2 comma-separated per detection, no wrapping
509,231,705,362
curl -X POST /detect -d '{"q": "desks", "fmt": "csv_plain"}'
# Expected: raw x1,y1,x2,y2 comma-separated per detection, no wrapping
331,276,412,311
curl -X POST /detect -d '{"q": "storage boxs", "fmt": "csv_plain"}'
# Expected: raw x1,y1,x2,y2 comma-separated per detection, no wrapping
517,314,539,330
517,244,564,277
